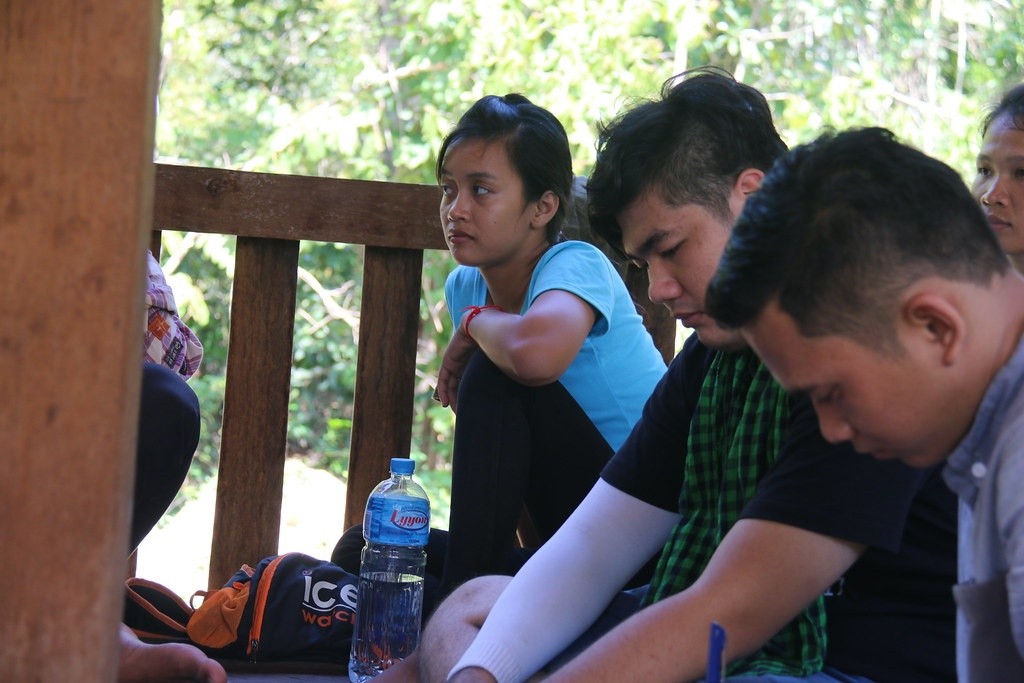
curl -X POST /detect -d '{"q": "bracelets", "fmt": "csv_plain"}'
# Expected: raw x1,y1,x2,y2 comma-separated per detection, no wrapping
464,304,504,344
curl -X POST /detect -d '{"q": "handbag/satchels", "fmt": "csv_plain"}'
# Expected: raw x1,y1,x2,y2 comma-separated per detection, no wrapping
123,552,359,676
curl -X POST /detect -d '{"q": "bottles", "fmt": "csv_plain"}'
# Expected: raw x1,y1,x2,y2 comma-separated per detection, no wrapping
347,458,431,683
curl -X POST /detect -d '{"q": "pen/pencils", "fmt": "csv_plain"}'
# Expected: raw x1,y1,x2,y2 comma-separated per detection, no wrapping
706,621,728,683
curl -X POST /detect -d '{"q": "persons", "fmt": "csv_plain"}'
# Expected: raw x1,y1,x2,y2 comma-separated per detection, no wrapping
414,71,960,683
705,124,1023,683
117,245,230,683
327,90,668,683
966,81,1023,284
